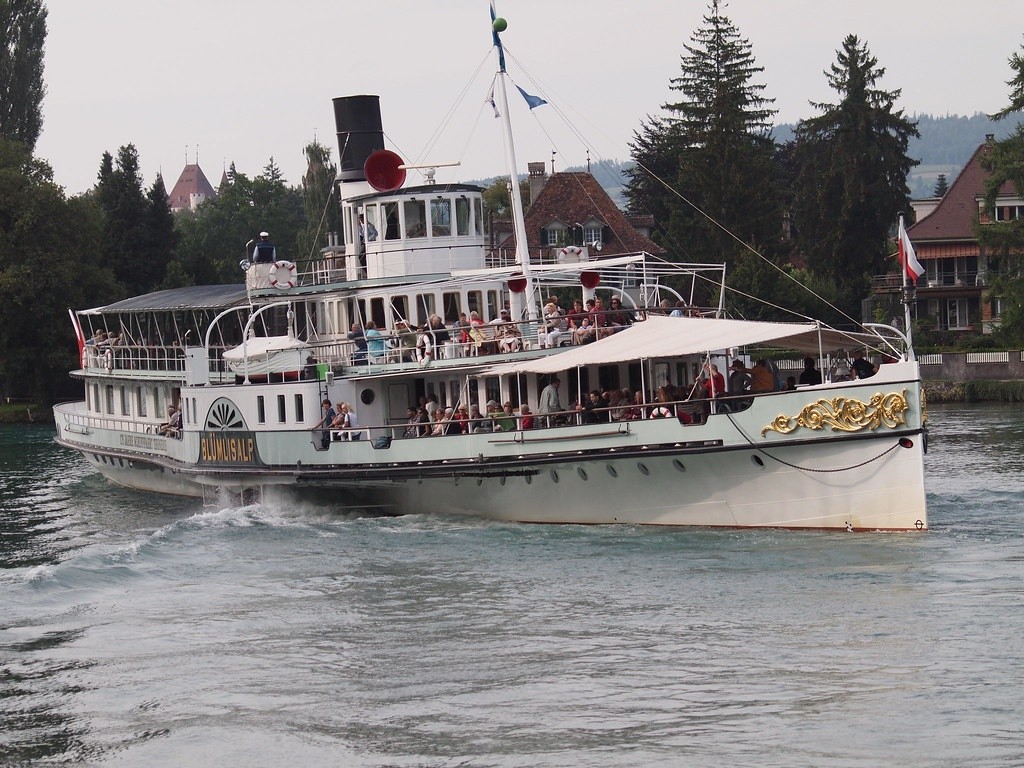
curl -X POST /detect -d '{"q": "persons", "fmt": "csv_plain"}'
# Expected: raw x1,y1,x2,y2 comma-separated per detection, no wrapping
357,214,378,273
573,350,898,424
86,328,183,440
347,295,706,362
312,399,362,449
253,231,277,264
403,377,565,439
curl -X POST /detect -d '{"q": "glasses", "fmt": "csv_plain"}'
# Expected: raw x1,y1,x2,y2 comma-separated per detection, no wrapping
612,302,617,304
419,412,423,414
460,409,464,411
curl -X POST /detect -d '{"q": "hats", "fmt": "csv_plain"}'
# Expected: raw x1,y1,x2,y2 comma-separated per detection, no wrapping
486,400,497,406
500,309,507,313
400,320,410,324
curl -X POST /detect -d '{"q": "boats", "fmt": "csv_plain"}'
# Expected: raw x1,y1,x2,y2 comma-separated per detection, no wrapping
53,1,928,531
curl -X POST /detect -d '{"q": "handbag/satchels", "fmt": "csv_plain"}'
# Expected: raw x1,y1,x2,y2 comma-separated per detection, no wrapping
337,424,351,438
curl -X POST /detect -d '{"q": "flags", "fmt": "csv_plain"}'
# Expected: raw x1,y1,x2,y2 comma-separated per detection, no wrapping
76,319,86,368
899,224,926,285
